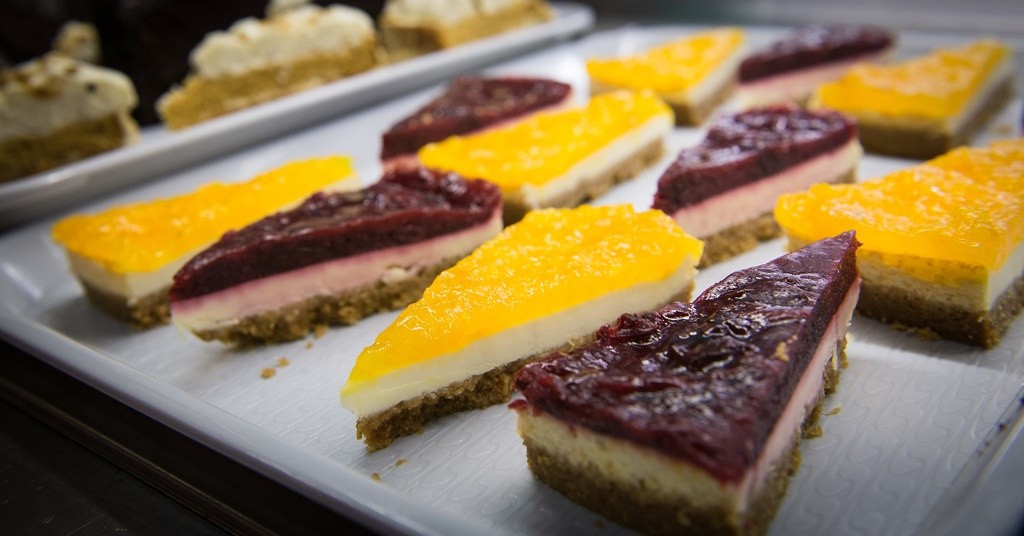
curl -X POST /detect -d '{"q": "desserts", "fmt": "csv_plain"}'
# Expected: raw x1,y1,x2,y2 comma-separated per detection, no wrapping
0,0,1024,536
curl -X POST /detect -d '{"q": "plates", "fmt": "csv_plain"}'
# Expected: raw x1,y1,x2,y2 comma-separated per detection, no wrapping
0,29,1024,536
0,0,596,230
